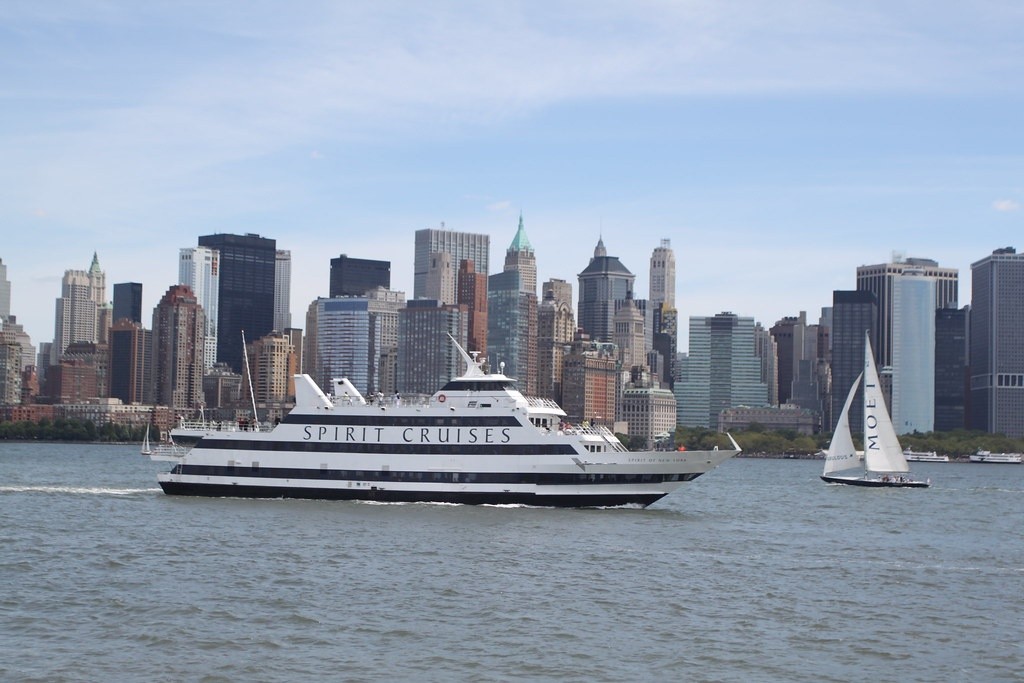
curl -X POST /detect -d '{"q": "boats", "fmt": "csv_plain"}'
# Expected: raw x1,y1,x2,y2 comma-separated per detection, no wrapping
151,330,742,512
150,431,188,461
970,447,1023,464
902,449,949,463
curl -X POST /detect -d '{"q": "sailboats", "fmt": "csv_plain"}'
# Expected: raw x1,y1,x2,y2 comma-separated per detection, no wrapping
818,328,934,485
140,422,152,454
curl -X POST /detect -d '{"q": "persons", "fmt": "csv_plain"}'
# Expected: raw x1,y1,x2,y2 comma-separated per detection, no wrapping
239,417,256,432
565,421,572,435
344,392,352,406
591,418,595,428
376,391,384,407
274,416,281,426
216,421,221,431
393,390,400,404
558,419,564,431
678,443,686,451
864,469,913,484
365,391,374,405
326,391,331,401
